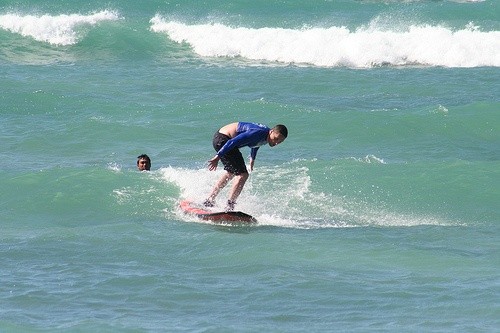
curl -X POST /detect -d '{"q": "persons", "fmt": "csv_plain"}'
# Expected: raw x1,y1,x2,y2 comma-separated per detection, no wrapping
136,153,152,171
203,121,289,212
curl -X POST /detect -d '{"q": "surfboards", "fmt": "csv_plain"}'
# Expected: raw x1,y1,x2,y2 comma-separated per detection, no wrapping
177,200,256,224
